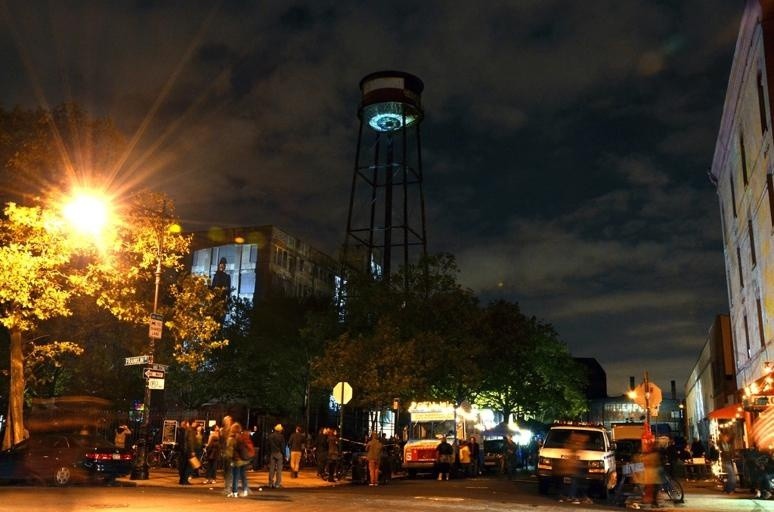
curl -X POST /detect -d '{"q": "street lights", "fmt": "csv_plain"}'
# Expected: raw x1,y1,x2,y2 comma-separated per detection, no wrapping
678,403,684,418
63,180,166,479
206,236,244,287
625,367,651,428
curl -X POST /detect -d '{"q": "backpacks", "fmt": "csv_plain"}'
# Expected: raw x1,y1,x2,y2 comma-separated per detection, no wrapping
231,434,256,461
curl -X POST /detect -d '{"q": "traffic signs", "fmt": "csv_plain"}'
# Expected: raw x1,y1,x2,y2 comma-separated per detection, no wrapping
149,313,162,339
123,355,148,366
142,366,164,379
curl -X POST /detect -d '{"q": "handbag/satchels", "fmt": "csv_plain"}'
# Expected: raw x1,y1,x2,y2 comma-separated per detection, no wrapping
198,470,208,477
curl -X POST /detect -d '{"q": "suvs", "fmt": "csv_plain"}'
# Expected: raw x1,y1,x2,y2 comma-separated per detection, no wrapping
535,420,617,497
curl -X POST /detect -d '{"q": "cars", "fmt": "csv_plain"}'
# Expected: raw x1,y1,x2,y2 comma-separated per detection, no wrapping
0,431,132,488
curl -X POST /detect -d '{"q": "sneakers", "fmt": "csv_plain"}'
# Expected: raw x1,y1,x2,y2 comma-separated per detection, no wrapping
227,492,238,497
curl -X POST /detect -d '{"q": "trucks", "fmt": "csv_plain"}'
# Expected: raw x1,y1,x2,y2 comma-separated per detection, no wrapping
402,400,483,478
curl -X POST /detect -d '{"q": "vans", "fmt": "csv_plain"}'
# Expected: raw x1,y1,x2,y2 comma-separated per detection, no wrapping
482,438,518,467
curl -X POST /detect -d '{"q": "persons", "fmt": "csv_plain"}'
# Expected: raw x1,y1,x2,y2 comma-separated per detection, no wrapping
252,424,542,491
284,425,308,476
218,412,239,495
194,423,204,442
179,418,200,484
363,431,383,487
266,424,284,488
224,422,250,499
433,436,454,483
108,418,131,451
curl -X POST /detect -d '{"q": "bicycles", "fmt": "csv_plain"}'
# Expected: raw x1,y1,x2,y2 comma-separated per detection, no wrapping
320,451,352,481
607,450,684,503
145,443,224,474
